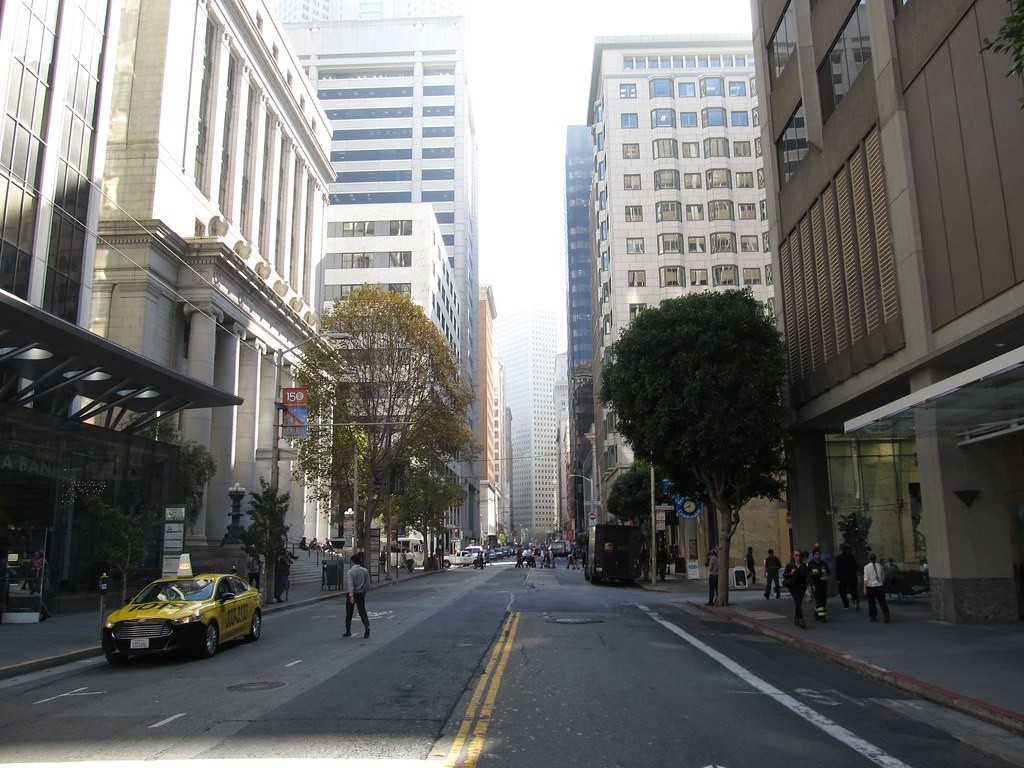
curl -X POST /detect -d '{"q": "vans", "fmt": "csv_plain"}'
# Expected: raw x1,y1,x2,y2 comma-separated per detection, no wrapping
465,546,484,559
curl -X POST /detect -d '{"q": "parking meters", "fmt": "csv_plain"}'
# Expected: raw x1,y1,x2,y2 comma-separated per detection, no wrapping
98,573,109,639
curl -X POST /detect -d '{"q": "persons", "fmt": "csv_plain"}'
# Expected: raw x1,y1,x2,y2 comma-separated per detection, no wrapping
28,552,43,595
705,546,718,606
299,536,342,553
404,548,415,572
783,548,829,629
832,543,928,624
567,547,587,569
746,547,756,584
342,554,370,638
474,551,492,569
321,560,330,591
515,543,555,569
764,549,782,600
247,539,293,604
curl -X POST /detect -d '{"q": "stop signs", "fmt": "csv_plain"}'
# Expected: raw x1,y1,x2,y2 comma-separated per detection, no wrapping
589,513,596,520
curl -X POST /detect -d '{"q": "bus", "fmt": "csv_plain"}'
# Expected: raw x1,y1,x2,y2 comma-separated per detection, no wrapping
379,538,425,567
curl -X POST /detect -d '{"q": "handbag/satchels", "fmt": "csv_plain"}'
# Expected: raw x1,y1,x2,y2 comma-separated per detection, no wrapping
781,575,796,587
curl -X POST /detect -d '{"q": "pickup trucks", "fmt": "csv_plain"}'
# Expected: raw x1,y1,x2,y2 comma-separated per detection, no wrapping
444,551,478,568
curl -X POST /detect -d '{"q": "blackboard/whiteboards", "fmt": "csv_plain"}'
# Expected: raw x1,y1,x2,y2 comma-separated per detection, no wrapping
676,557,686,574
734,570,748,588
687,562,699,580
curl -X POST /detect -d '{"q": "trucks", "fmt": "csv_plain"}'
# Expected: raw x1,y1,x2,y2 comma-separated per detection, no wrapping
585,524,642,584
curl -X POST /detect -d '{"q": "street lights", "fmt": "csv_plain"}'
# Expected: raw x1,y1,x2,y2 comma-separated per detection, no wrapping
569,474,593,513
266,333,350,609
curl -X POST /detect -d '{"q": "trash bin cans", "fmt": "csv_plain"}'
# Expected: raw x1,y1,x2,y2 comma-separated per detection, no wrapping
434,558,440,569
321,560,344,591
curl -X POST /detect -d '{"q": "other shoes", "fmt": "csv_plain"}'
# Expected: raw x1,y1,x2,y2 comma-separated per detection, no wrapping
853,600,859,611
21,587,27,590
343,632,351,636
870,618,878,622
764,593,769,599
813,611,827,623
841,607,849,609
795,618,805,628
884,612,890,623
364,626,370,638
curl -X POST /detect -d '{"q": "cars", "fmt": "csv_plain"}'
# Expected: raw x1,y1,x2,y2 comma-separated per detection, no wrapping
514,548,520,556
494,548,503,559
489,550,497,561
102,554,261,663
501,547,513,557
